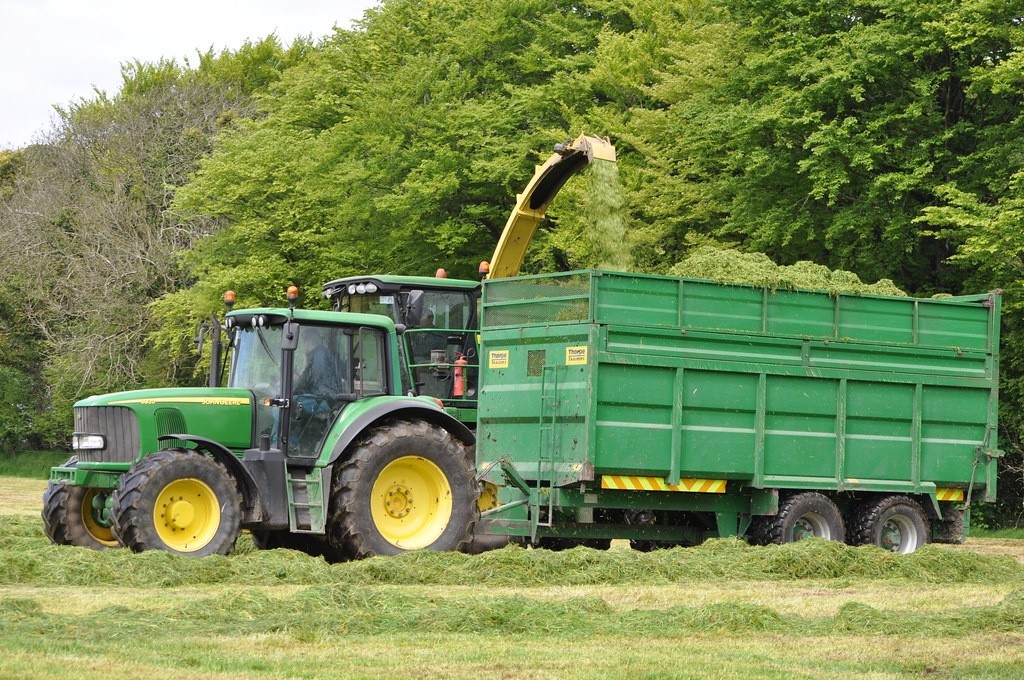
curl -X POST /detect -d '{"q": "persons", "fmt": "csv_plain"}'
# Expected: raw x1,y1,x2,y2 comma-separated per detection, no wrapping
257,328,348,455
410,308,447,363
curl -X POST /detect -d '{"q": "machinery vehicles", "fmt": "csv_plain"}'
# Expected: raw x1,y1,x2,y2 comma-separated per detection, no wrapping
321,131,616,404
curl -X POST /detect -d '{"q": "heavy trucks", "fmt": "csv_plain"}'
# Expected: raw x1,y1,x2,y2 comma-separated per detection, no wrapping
40,271,1007,566
